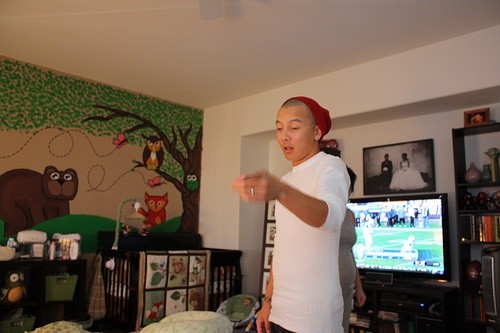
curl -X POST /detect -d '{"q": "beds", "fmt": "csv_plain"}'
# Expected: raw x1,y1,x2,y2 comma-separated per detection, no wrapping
97,248,244,333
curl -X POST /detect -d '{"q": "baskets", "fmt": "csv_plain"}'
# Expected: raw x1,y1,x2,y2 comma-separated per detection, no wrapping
0,315,35,333
44,275,78,303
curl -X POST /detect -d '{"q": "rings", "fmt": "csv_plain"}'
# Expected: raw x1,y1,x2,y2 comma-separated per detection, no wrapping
250,188,254,194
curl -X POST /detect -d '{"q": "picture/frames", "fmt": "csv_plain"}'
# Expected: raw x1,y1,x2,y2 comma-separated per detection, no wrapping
464,107,491,128
363,138,436,195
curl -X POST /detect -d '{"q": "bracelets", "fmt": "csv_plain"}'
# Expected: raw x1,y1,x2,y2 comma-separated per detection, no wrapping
265,297,271,302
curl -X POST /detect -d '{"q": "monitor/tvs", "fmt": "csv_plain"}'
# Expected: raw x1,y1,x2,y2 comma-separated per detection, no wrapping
347,193,450,286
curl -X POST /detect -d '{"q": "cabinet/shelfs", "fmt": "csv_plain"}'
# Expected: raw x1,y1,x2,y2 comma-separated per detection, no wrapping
452,122,500,333
0,257,86,333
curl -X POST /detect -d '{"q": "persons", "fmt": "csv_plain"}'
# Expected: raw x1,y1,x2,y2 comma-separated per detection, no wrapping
339,166,366,333
232,96,351,333
378,153,393,192
390,153,429,191
376,202,429,229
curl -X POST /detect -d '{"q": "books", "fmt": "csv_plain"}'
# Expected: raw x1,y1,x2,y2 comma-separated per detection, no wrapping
472,214,500,242
464,296,485,321
491,158,498,182
351,315,415,333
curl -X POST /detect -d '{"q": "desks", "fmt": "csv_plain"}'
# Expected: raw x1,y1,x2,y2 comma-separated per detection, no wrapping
347,279,458,333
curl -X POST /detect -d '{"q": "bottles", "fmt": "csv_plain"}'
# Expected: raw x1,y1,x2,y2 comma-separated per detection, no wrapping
465,163,492,183
49,239,79,260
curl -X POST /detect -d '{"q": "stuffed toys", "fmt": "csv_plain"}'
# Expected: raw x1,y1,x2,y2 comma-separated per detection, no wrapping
0,269,27,306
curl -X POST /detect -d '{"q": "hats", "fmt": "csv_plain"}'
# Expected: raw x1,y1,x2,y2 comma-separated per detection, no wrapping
284,97,331,143
